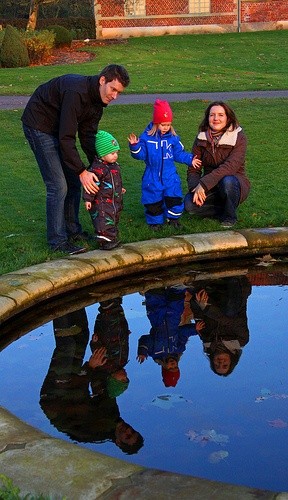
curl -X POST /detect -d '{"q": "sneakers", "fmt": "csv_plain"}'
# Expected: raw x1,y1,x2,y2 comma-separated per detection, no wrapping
220,220,237,227
71,231,96,242
53,243,87,255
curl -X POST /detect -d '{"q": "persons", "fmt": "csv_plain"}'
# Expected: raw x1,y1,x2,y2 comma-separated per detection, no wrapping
21,64,130,255
128,99,202,232
136,284,206,388
82,130,127,250
184,101,250,227
90,291,132,400
188,275,252,377
39,306,145,455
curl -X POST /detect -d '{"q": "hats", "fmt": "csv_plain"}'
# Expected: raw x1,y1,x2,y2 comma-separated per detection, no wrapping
152,99,172,123
95,130,120,158
106,376,129,399
162,367,180,387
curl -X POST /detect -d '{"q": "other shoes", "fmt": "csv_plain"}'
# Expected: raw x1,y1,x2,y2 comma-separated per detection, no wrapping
116,239,123,247
98,242,117,250
168,222,181,228
99,299,115,309
148,224,164,232
